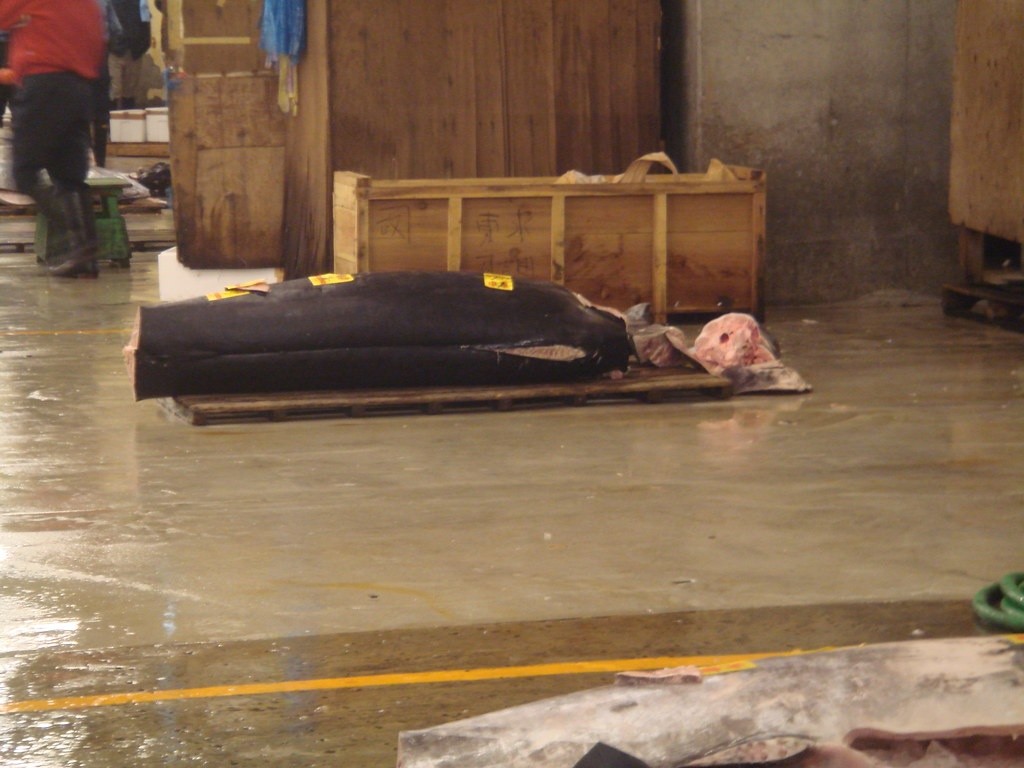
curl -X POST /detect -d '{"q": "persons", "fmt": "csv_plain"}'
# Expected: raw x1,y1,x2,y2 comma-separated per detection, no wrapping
0,0,152,277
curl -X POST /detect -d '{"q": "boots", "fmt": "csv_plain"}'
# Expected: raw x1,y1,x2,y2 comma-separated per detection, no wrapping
48,192,100,279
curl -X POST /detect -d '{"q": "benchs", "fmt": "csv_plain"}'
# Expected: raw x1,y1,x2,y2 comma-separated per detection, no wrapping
1,168,137,268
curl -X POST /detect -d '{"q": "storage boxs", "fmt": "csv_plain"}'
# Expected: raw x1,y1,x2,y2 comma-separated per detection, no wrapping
331,160,766,326
146,107,171,143
109,107,147,144
